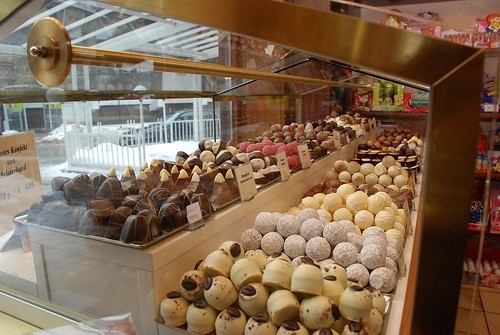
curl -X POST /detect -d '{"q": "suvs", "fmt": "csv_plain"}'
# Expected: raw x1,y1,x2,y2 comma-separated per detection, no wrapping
118,107,220,147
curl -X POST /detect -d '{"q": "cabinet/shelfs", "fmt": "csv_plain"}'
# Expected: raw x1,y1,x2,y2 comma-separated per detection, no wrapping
347,47,500,291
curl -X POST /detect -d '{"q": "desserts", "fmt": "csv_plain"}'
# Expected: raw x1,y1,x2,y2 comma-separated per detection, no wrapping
27,113,423,335
